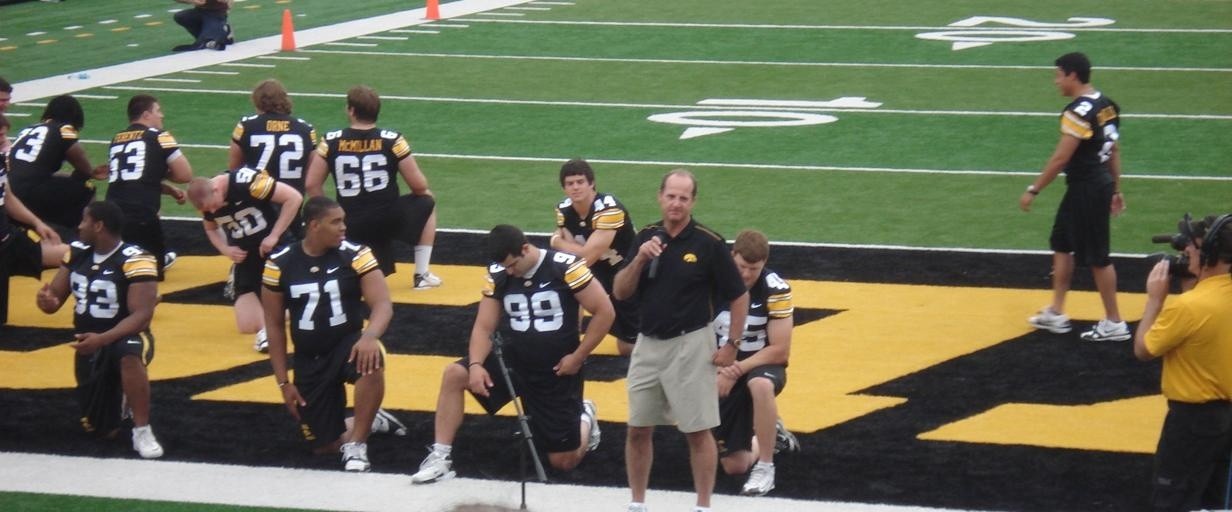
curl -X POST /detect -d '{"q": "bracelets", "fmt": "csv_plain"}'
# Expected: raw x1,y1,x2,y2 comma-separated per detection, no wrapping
1024,185,1039,196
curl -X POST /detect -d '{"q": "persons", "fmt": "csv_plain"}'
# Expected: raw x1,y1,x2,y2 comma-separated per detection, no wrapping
186,167,306,349
35,201,164,459
229,82,320,193
1020,53,1134,343
262,193,409,476
412,157,802,511
1,75,193,320
1130,215,1232,511
306,84,443,290
173,1,235,53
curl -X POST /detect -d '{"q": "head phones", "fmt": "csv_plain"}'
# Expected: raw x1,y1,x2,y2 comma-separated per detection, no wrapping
1199,213,1232,269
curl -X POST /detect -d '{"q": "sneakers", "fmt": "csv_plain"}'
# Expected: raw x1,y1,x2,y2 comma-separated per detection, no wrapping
412,450,456,484
740,462,776,497
339,441,372,472
252,328,269,352
412,270,443,288
368,407,408,437
582,399,601,454
1027,307,1074,336
131,422,164,459
774,418,800,456
1079,319,1132,342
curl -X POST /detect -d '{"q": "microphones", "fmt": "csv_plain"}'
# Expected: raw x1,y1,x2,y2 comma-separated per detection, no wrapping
647,225,667,278
